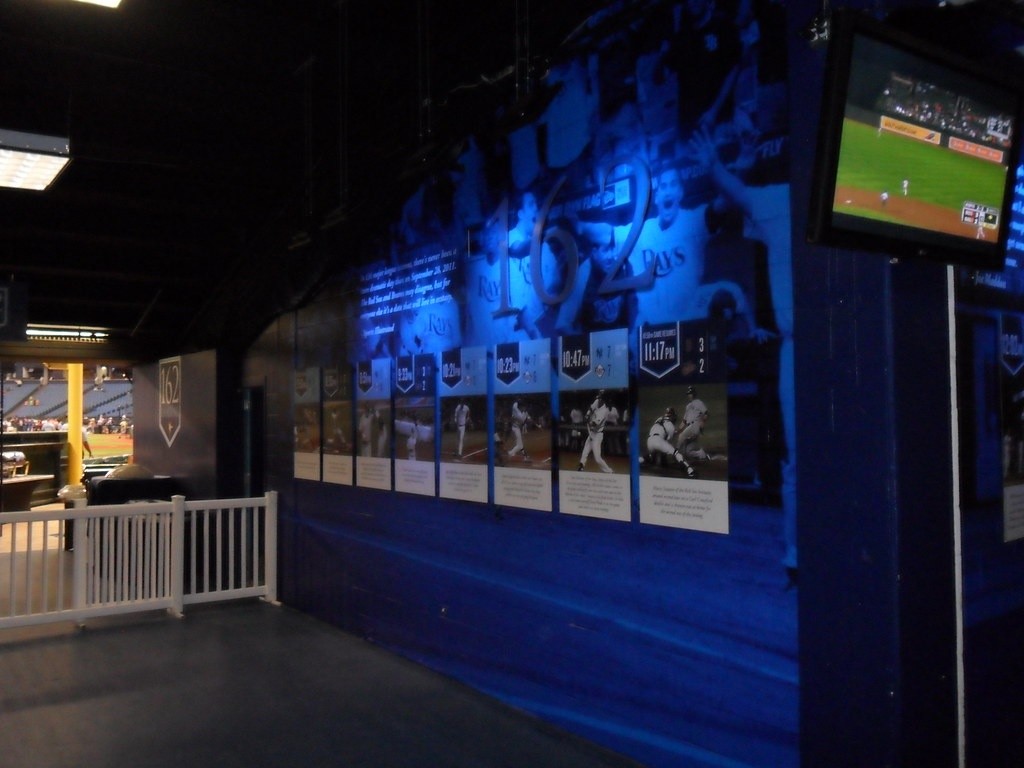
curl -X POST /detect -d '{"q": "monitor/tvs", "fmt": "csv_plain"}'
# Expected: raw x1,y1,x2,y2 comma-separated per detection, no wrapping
802,5,1024,271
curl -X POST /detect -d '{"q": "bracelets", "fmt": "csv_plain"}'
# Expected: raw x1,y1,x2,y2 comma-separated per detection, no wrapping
468,416,469,417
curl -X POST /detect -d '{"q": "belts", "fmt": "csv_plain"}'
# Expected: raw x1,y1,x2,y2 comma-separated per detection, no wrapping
687,420,696,426
459,425,464,427
648,434,660,437
591,429,603,433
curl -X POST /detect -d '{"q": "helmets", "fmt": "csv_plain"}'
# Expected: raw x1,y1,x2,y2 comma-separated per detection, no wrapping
684,387,695,395
665,407,674,415
595,390,606,400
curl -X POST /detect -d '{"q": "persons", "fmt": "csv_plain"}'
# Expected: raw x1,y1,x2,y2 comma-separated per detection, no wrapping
903,178,908,195
359,407,388,458
881,191,888,206
678,338,697,398
94,373,104,391
24,396,40,406
331,408,347,445
643,386,711,477
896,82,1003,145
4,415,62,432
393,1,795,352
977,226,985,238
407,416,421,461
508,398,542,461
561,389,630,473
688,123,797,583
454,399,470,456
84,415,130,435
62,413,93,458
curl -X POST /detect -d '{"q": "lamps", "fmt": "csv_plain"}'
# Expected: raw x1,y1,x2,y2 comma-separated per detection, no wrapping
0,128,73,193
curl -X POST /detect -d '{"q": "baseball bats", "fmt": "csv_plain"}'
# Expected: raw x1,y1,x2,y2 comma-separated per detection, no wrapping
586,422,593,441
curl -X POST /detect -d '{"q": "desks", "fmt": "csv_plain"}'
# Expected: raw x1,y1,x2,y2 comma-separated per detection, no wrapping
1,474,54,512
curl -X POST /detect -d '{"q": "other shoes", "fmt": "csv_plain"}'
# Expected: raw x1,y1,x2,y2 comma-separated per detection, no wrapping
577,463,584,471
520,449,526,455
705,452,711,461
689,468,695,477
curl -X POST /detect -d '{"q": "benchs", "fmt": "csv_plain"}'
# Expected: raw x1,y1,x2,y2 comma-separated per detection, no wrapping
560,424,629,456
